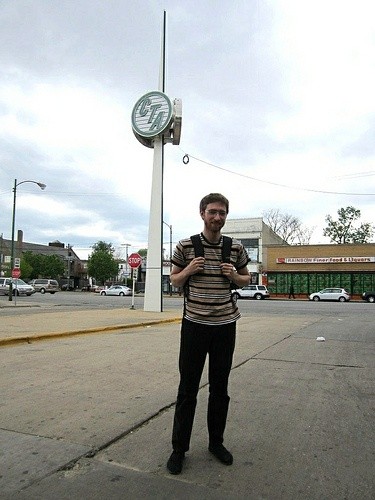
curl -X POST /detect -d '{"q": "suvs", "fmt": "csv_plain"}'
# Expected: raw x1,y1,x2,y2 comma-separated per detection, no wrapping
231,284,270,300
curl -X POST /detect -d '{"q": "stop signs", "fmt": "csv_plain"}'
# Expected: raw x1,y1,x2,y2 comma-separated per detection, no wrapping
128,253,143,268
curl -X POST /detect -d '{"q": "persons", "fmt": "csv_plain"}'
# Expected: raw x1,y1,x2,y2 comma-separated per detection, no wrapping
289,283,295,299
62,283,68,291
86,283,91,291
167,193,251,474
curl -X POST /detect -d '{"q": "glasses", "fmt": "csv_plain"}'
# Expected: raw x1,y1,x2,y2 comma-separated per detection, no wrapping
207,209,225,215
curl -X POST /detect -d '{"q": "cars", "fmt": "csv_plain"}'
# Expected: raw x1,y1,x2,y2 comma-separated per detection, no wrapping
61,283,104,293
100,285,133,296
362,290,375,303
0,277,35,296
309,287,351,302
27,278,59,294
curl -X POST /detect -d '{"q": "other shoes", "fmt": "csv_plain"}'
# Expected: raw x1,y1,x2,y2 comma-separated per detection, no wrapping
167,452,185,475
208,443,233,466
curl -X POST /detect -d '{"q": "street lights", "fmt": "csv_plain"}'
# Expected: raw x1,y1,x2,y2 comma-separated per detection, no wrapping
8,179,47,301
